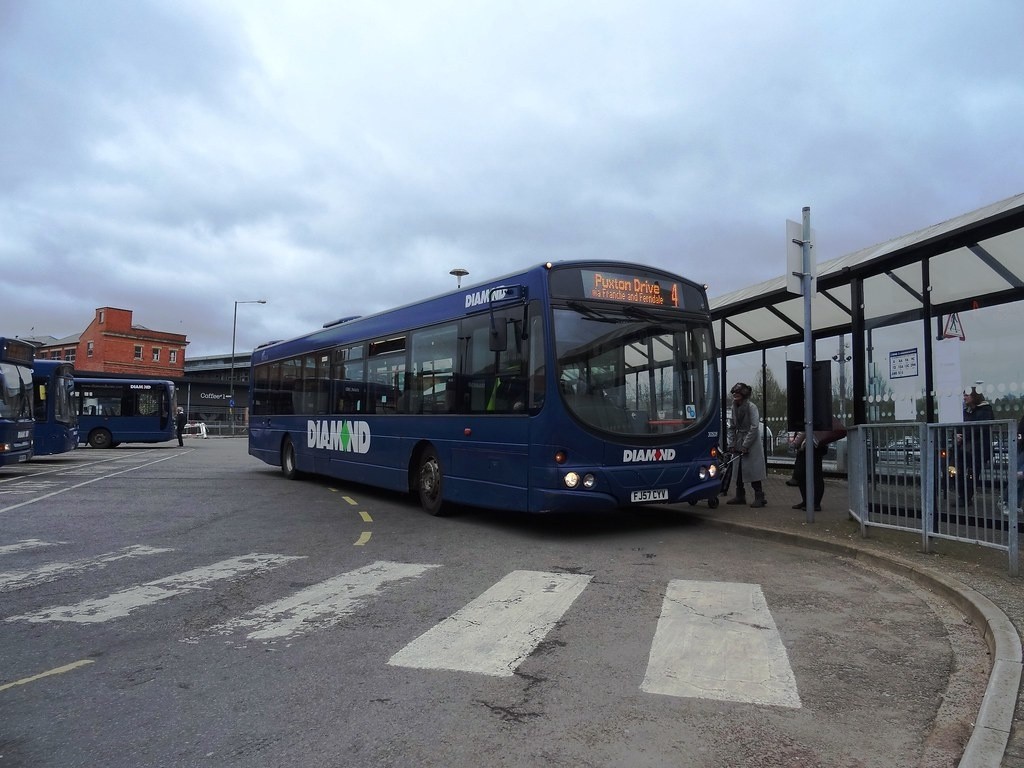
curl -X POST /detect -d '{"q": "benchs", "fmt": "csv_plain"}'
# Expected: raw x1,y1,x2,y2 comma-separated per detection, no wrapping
868,447,1010,499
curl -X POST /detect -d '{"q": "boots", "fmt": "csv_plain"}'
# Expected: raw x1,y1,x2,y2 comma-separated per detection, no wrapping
750,491,767,507
727,488,746,504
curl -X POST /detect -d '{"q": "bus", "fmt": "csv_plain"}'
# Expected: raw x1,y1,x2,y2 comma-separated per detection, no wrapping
0,335,35,472
30,358,81,458
247,259,726,518
72,377,179,449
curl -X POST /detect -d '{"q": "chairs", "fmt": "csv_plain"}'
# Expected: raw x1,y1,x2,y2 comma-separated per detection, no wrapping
90,405,116,416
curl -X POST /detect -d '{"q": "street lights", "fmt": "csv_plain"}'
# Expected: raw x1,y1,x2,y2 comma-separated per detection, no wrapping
230,300,266,403
449,268,469,289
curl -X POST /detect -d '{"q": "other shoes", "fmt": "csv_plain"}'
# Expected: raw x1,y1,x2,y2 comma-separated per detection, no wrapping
1017,507,1023,514
801,504,821,510
786,478,800,486
997,496,1010,516
792,501,805,509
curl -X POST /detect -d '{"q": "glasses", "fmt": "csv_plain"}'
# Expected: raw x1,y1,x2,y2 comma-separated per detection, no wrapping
731,389,741,394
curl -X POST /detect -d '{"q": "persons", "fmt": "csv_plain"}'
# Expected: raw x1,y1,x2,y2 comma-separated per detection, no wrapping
397,360,573,414
785,431,828,510
727,383,767,507
950,386,1024,518
175,407,187,447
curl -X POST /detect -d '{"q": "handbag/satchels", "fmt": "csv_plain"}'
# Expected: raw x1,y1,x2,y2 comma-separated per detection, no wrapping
813,414,848,448
